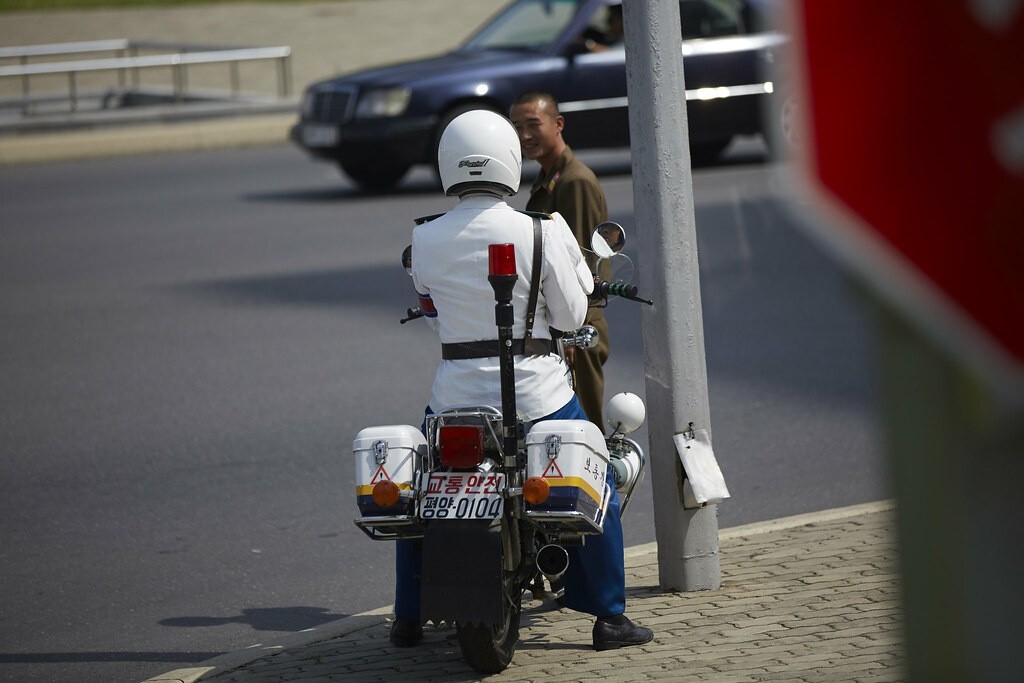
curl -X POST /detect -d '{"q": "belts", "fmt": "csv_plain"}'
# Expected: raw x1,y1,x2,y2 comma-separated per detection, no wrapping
442,338,554,360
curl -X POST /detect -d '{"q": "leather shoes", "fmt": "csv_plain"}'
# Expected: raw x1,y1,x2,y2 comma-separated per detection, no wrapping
389,618,423,647
593,619,654,651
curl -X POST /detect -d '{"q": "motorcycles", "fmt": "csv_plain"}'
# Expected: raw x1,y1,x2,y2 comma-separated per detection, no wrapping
351,219,654,674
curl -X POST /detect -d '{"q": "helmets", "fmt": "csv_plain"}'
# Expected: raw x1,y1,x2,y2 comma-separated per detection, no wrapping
436,111,523,195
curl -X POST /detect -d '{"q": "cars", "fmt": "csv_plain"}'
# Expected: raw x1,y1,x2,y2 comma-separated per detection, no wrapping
281,0,798,199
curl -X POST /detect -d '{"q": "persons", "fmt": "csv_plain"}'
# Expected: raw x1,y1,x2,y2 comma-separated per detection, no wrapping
597,224,622,249
389,110,654,650
579,3,624,53
510,94,610,436
